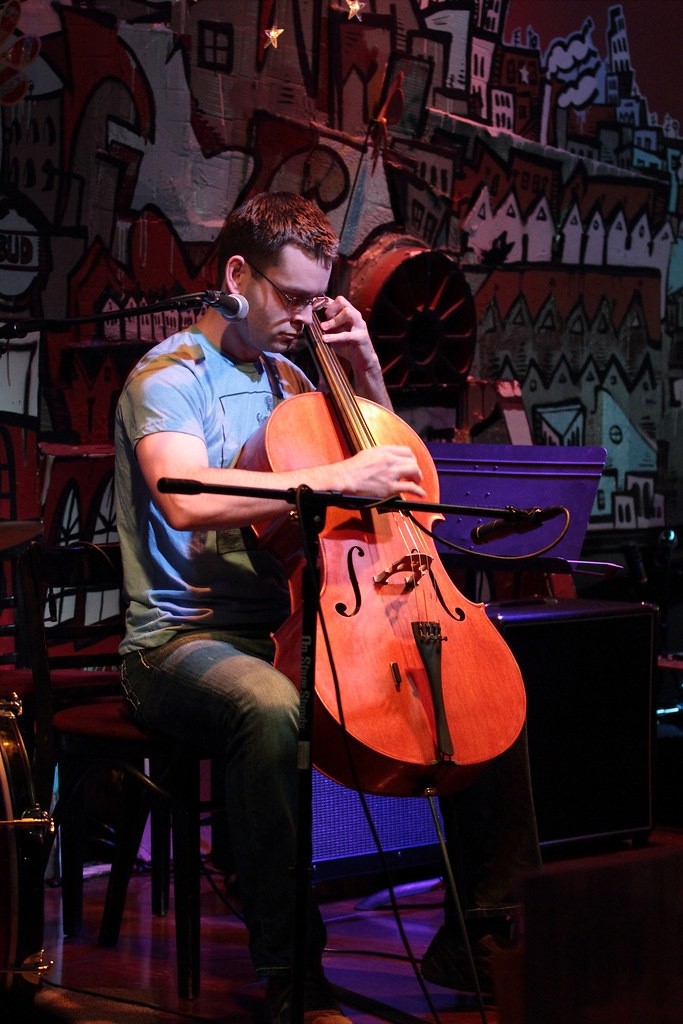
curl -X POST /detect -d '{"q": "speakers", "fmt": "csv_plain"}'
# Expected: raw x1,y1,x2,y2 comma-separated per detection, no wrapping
478,594,661,860
209,758,448,905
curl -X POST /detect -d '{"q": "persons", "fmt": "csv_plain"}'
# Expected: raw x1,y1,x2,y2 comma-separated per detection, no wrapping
114,192,525,1024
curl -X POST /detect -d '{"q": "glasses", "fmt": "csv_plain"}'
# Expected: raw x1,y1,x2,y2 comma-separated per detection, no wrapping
244,258,329,312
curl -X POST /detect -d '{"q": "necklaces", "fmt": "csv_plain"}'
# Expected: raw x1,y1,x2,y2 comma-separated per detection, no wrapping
199,341,260,374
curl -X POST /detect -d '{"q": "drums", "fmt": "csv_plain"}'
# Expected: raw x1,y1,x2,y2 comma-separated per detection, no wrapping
0,690,57,1024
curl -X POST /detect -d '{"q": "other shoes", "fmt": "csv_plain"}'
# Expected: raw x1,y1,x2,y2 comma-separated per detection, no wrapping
420,950,493,994
303,1008,352,1024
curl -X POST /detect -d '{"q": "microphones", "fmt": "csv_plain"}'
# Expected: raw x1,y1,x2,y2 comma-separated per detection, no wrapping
471,507,563,545
202,289,250,324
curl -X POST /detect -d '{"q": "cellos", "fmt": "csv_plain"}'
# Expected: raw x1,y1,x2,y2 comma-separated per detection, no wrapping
235,306,528,1024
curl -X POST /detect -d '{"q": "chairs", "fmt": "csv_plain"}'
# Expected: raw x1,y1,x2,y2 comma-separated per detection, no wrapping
10,541,229,1000
